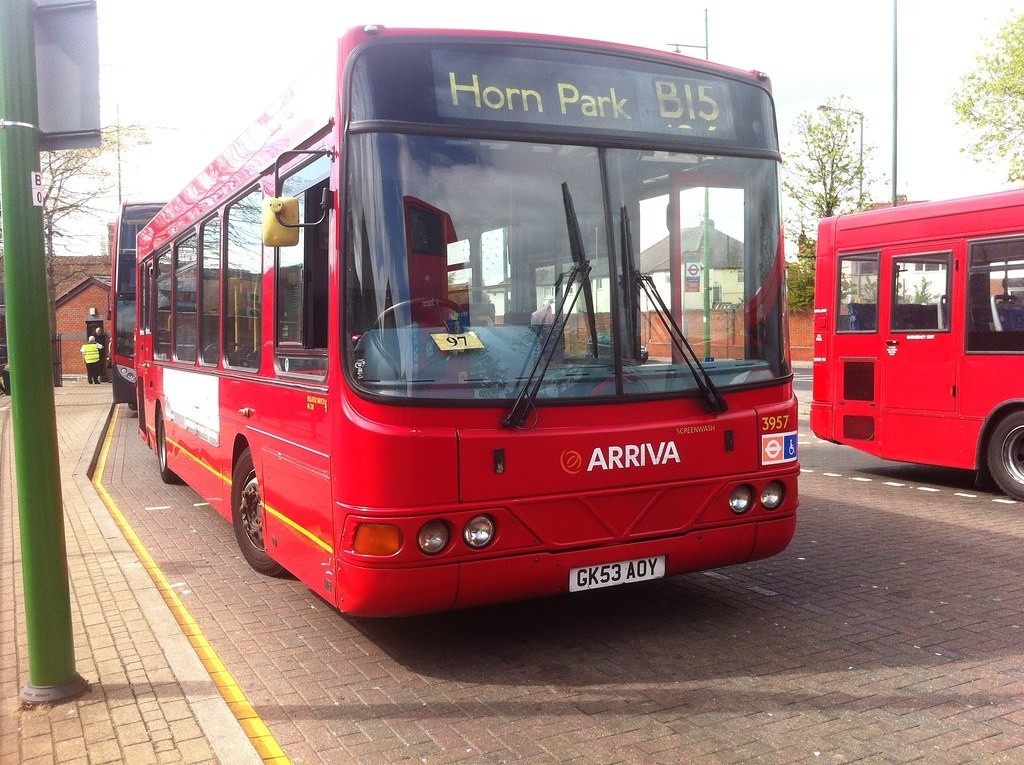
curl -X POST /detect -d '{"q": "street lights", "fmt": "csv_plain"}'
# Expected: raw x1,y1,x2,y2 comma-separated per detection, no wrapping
818,104,864,214
668,43,712,362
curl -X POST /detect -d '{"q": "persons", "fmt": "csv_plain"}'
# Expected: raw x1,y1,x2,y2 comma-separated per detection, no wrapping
94,327,109,382
80,336,103,384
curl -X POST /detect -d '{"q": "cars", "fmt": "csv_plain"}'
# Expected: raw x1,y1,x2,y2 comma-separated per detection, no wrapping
587,330,648,363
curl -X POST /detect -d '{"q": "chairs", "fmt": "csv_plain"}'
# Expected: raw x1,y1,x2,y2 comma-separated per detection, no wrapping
848,294,1024,333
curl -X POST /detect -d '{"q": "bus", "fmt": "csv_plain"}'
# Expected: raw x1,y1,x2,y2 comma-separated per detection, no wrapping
134,27,800,620
809,189,1024,501
108,204,168,410
